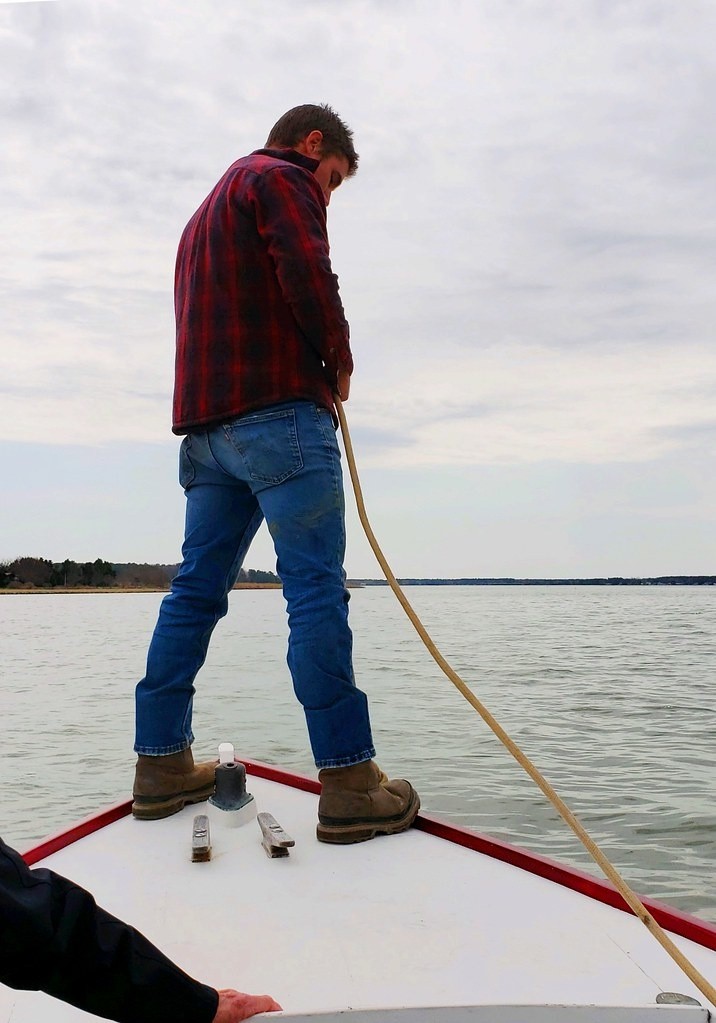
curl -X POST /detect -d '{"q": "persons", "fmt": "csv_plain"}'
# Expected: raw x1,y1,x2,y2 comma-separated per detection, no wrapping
130,104,419,845
0,837,283,1023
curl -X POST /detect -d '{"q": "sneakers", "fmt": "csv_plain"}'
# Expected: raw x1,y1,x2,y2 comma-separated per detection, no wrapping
131,749,219,822
317,760,420,846
